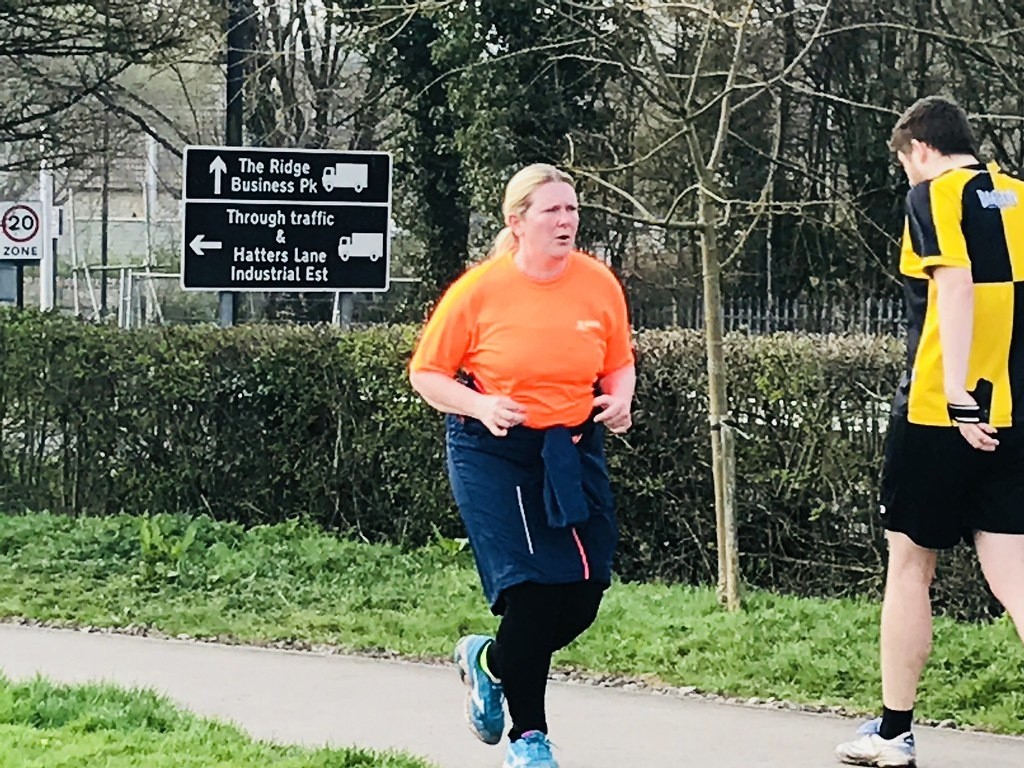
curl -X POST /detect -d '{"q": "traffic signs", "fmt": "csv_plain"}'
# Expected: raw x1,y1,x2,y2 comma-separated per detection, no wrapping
179,144,393,293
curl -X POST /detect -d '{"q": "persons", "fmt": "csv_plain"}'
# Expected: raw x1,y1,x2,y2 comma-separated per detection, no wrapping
408,163,636,768
835,95,1024,768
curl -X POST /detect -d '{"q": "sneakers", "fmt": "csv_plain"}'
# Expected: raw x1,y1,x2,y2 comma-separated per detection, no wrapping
503,729,559,768
833,731,917,768
453,634,505,745
